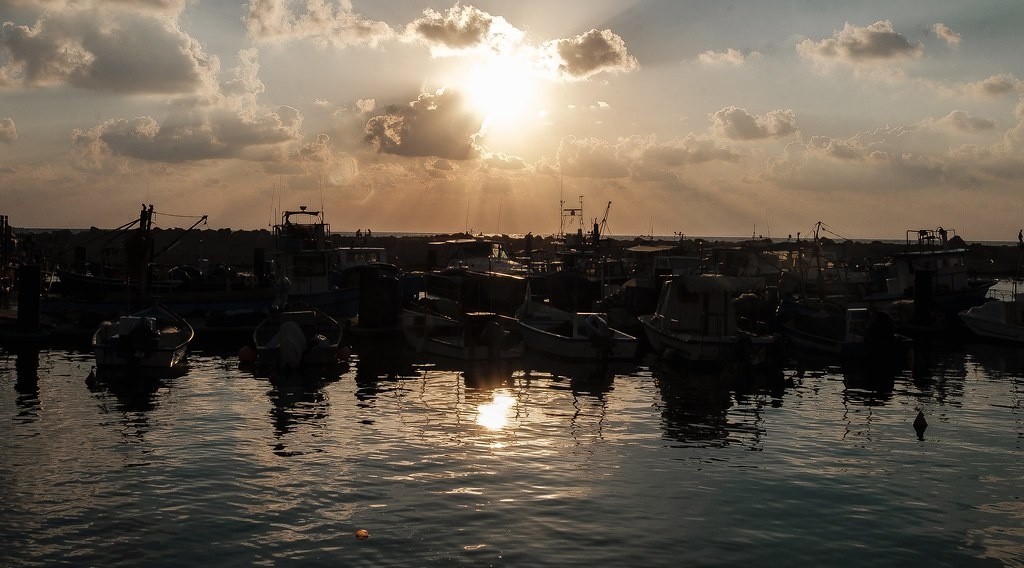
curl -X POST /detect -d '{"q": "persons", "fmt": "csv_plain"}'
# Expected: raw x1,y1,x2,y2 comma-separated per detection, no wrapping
356,229,360,237
368,229,372,236
277,272,291,303
1016,229,1023,245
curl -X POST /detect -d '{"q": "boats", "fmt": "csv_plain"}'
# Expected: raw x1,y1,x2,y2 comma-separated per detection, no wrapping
775,295,915,363
956,280,1024,345
398,298,527,360
636,272,778,366
89,299,196,370
512,296,643,363
1,193,997,330
252,299,345,366
